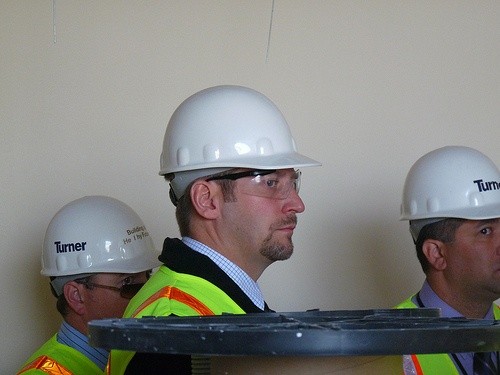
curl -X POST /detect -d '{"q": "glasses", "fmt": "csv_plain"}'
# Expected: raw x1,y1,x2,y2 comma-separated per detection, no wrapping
210,170,301,200
73,280,147,300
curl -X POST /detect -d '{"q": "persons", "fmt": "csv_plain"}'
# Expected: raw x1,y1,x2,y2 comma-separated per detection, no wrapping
368,144,500,374
7,196,165,375
104,85,326,373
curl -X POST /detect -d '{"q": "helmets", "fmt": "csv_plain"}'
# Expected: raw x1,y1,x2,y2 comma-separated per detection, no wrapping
40,195,164,277
399,144,500,220
158,85,322,179
